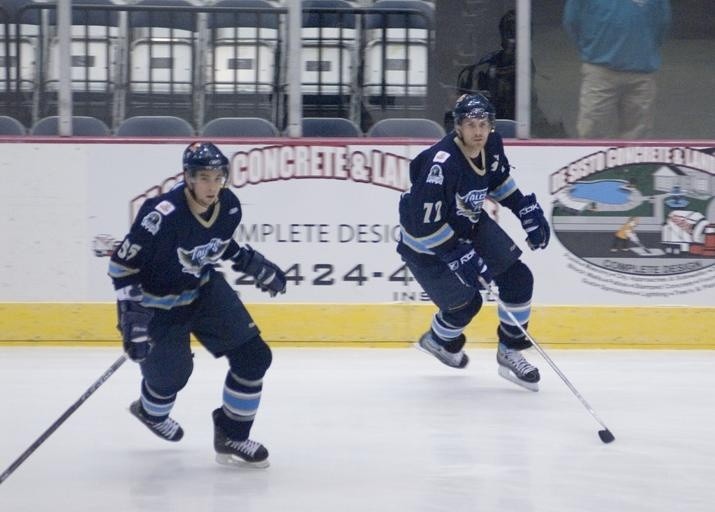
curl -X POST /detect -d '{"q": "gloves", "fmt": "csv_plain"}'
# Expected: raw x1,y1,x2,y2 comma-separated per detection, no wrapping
115,300,155,362
444,239,494,291
236,246,287,295
512,195,552,250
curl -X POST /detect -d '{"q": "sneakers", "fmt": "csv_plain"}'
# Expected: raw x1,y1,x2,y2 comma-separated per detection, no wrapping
494,343,540,383
417,331,470,369
129,396,184,442
211,420,270,462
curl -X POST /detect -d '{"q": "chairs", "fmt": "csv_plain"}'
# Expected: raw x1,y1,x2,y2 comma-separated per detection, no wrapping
1,1,520,144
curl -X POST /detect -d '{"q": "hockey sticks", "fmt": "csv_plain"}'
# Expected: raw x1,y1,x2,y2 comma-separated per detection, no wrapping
479,278,615,443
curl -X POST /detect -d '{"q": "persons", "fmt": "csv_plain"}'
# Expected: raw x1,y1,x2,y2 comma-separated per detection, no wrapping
106,139,287,462
456,9,549,139
396,91,551,384
609,213,642,252
563,0,674,139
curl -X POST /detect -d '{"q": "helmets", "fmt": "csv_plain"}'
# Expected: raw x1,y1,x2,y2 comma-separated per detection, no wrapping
182,140,233,206
452,92,496,148
